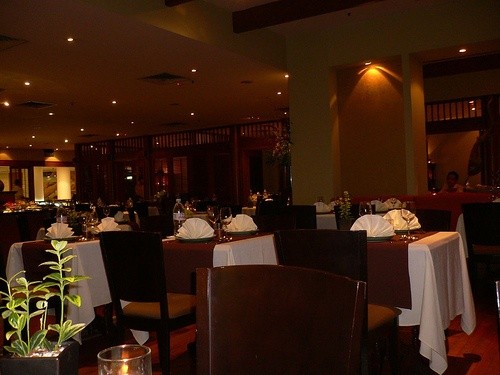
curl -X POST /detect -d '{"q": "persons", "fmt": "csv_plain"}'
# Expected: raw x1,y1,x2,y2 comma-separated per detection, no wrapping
13,179,22,199
441,171,463,192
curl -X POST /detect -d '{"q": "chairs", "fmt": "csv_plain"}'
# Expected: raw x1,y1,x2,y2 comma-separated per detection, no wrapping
0,211,46,257
97,229,197,375
254,204,315,231
333,203,375,229
462,201,500,298
414,207,452,231
274,228,403,375
195,261,370,375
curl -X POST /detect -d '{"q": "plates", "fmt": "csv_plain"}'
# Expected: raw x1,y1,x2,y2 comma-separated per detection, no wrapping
317,210,333,214
175,235,217,240
367,235,393,242
225,230,257,236
394,230,416,234
44,236,79,243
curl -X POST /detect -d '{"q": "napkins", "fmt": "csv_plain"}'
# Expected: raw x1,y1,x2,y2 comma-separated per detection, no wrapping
383,209,421,230
368,197,402,212
226,213,258,232
96,216,121,232
176,217,215,238
350,214,394,236
45,222,74,238
313,201,343,212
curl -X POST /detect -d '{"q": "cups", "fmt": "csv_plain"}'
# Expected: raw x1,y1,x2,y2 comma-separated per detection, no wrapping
359,202,372,216
97,344,153,375
56,210,67,224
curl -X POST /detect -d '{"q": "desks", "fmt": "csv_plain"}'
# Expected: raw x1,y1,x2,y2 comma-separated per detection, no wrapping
7,238,132,347
160,229,478,375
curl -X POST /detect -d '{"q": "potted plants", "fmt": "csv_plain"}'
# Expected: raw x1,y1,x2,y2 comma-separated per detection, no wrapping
0,238,94,375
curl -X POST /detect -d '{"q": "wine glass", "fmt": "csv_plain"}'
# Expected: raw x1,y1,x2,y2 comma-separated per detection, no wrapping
207,207,219,229
400,201,419,240
391,192,399,210
102,206,110,216
87,213,99,240
219,206,232,242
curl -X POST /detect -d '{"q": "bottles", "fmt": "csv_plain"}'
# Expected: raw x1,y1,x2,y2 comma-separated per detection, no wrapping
172,198,186,237
264,188,267,198
